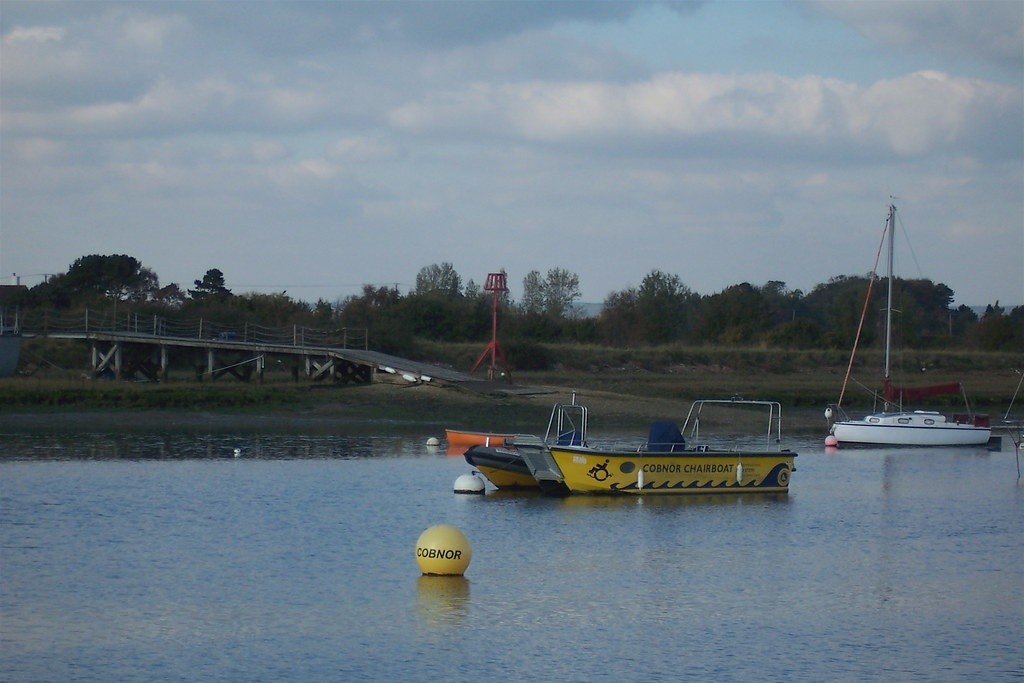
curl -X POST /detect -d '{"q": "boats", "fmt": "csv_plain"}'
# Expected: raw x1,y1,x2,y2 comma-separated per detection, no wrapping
463,391,798,494
443,427,519,446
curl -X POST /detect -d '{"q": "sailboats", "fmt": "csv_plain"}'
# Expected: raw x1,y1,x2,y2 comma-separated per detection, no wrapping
826,192,1024,451
